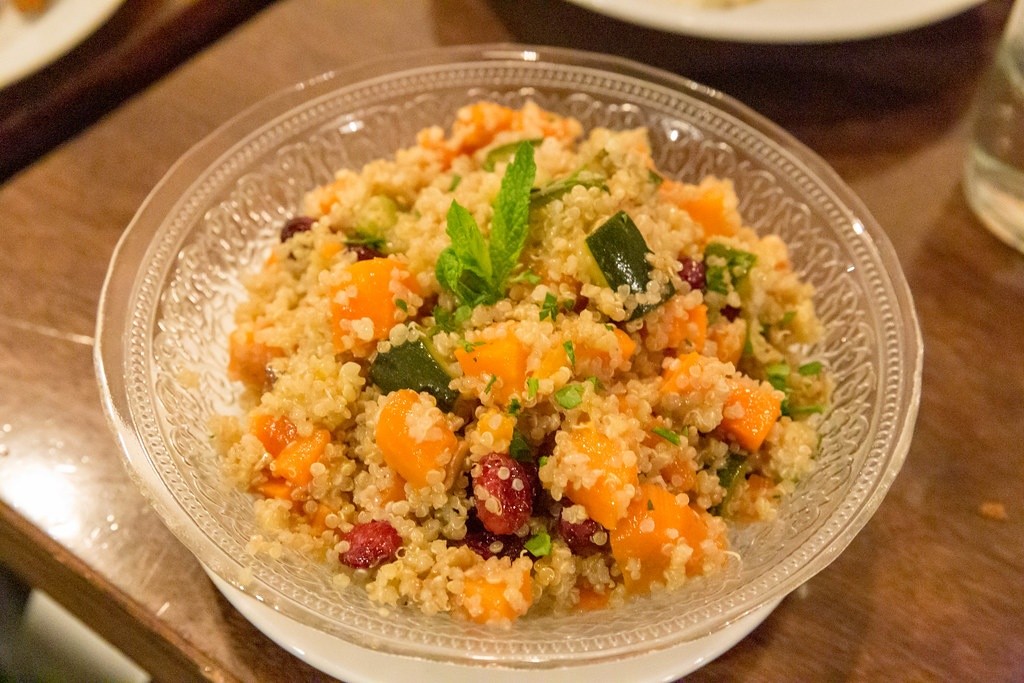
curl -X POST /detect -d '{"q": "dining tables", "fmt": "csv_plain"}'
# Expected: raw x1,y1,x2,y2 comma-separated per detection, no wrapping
0,0,1024,683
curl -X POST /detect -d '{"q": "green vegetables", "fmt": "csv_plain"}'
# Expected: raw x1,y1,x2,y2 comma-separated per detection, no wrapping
337,135,825,555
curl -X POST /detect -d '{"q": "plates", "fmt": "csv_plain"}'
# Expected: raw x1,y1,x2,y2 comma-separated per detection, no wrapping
570,1,992,46
1,0,124,93
190,559,789,683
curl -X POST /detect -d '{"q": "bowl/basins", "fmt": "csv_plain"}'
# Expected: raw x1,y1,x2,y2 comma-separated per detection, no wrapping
95,41,925,662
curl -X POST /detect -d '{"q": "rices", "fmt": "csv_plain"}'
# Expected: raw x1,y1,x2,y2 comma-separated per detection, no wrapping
215,104,836,628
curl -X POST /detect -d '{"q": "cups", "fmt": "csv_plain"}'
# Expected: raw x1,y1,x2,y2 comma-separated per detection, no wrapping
961,0,1024,254
